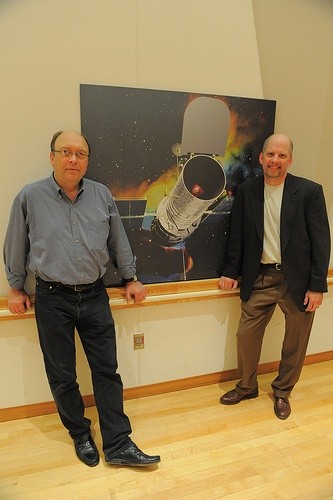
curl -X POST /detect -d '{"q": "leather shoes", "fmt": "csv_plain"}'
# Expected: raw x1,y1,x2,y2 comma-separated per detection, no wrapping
221,386,262,402
73,434,99,467
107,440,166,464
273,395,290,420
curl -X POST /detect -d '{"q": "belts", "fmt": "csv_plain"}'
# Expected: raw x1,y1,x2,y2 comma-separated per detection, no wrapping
258,264,289,272
34,279,106,292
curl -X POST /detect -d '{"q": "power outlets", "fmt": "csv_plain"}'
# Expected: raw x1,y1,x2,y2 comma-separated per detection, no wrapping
133,333,145,350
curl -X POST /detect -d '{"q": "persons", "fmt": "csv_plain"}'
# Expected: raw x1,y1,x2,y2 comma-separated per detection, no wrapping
4,131,161,466
218,132,331,420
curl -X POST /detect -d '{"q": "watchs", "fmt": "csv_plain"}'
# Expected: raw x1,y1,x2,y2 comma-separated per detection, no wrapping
126,276,138,282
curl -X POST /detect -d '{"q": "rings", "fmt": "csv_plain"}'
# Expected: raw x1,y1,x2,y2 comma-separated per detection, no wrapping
316,305,320,308
11,306,14,309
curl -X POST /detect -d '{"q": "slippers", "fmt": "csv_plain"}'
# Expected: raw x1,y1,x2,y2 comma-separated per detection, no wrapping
50,146,92,159
